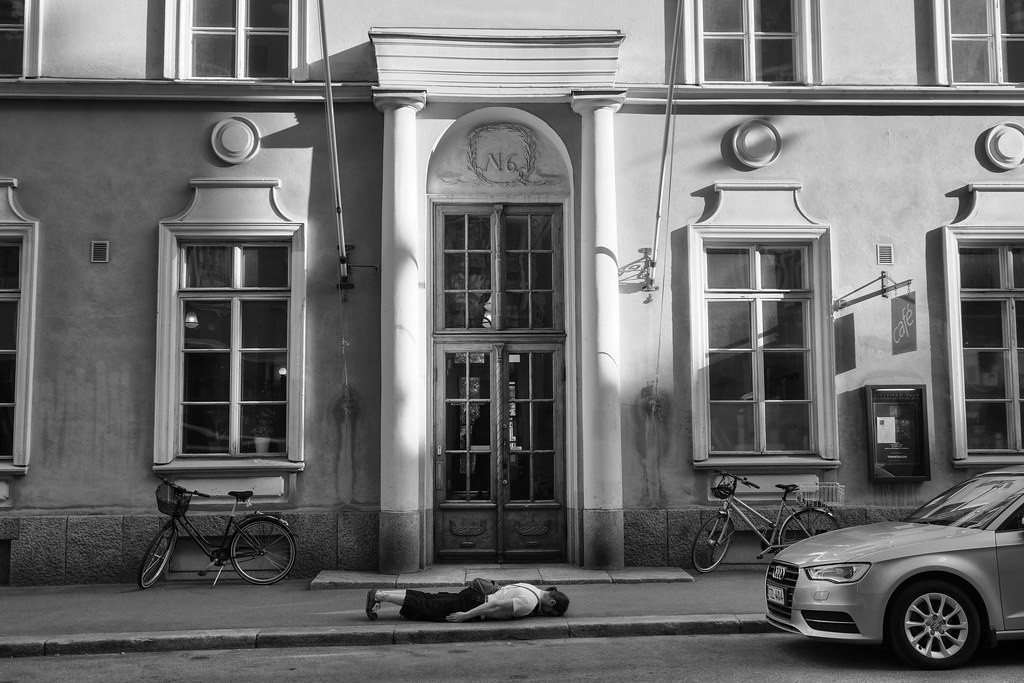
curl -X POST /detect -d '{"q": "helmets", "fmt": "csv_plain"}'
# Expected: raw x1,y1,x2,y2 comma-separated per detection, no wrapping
711,482,734,499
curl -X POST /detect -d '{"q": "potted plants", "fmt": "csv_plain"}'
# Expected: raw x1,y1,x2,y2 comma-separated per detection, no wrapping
254,413,270,453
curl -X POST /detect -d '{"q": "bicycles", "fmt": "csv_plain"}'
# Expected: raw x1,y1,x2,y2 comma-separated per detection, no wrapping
690,466,842,575
138,469,297,591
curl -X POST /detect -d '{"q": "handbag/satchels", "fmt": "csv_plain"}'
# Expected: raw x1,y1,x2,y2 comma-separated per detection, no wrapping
463,577,501,595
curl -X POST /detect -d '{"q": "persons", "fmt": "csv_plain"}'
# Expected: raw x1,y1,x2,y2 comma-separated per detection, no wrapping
365,582,570,623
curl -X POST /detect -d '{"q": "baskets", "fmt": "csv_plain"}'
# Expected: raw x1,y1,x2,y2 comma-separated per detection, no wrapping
156,483,193,518
796,480,846,509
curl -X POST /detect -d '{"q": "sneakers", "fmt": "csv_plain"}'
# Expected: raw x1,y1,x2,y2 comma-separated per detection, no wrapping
365,586,381,621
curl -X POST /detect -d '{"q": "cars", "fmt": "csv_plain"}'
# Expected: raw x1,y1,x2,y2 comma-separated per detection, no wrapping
764,463,1024,670
